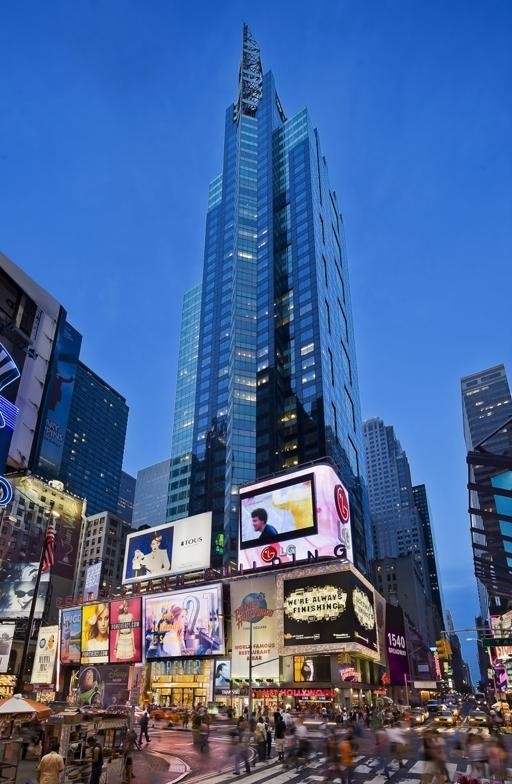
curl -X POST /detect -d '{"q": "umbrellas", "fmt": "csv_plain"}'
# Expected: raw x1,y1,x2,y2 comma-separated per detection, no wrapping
1,697,53,734
0,693,38,713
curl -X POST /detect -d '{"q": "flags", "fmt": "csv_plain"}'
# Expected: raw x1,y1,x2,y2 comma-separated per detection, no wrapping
41,511,59,573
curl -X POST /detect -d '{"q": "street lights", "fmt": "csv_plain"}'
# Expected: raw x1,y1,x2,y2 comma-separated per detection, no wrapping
466,638,498,702
248,607,287,735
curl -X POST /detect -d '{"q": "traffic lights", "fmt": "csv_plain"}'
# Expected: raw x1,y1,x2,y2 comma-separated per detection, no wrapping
436,640,452,659
338,653,351,666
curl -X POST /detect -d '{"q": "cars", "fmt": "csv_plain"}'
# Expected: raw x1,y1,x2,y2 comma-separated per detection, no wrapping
79,705,192,724
410,693,487,726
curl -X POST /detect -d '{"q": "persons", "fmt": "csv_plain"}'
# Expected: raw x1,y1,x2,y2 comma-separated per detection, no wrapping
70,723,84,741
111,697,118,710
6,581,45,613
300,662,312,682
215,662,230,688
113,599,137,662
63,620,71,656
134,532,171,578
180,696,508,784
106,752,125,784
82,603,108,663
88,591,94,601
119,749,134,783
138,712,152,744
21,723,37,760
159,605,187,656
127,727,140,751
251,507,279,539
83,736,104,784
36,740,65,784
206,615,221,636
